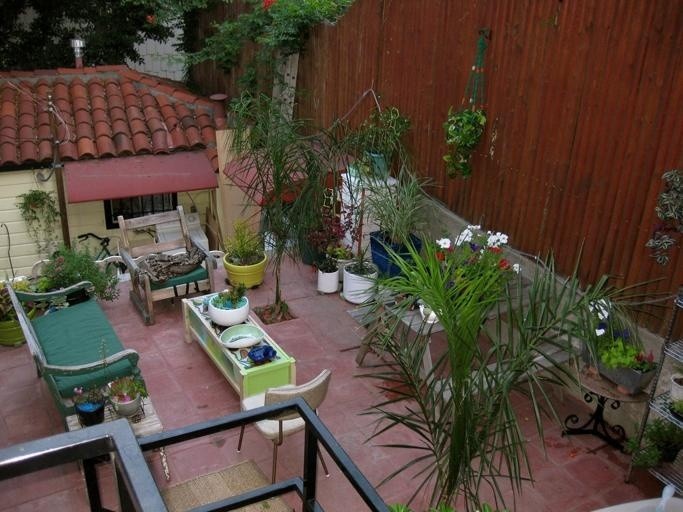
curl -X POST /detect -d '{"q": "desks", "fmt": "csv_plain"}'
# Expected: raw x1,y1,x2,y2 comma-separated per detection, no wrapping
561,374,670,452
354,258,555,387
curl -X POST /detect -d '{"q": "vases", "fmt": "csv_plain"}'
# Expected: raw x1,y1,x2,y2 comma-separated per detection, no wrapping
417,304,443,324
43,286,90,306
0,307,35,348
599,356,658,395
670,373,683,402
75,403,105,425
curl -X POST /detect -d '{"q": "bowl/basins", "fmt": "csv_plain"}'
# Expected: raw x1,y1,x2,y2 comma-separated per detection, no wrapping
218,323,267,350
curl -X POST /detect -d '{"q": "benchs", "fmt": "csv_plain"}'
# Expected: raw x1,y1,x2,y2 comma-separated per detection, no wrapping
434,335,582,423
5,277,148,433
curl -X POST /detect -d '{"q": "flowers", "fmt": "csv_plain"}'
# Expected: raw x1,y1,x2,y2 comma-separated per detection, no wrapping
1,277,33,315
38,244,118,302
436,224,522,285
71,386,105,404
595,320,656,372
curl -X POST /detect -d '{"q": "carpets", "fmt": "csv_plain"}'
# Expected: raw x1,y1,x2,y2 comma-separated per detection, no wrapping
159,459,294,512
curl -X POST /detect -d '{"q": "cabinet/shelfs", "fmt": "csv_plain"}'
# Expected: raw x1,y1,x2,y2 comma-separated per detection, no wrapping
624,287,683,499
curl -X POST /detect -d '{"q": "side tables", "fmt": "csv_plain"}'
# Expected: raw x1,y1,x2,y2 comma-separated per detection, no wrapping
66,395,170,500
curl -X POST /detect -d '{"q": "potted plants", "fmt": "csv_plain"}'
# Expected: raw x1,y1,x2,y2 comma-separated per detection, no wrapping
325,242,356,282
313,252,341,294
16,189,62,252
343,245,378,306
298,231,326,266
208,278,250,327
354,108,407,179
623,417,682,469
343,150,447,278
442,105,486,180
221,218,267,289
108,374,148,416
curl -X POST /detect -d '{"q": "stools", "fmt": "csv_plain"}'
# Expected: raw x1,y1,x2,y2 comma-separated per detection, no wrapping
347,293,381,329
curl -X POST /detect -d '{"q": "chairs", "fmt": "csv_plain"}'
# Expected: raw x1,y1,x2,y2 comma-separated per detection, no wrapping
237,368,335,485
117,205,217,326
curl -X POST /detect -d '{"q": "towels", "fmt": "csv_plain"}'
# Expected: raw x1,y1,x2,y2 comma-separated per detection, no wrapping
248,344,277,362
